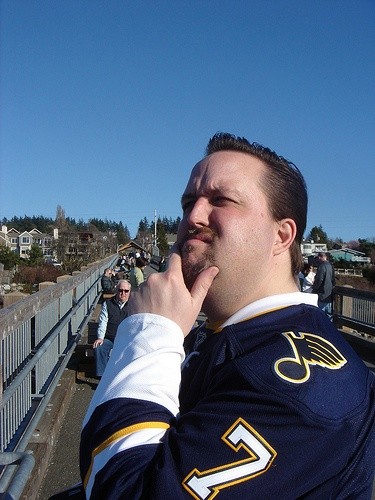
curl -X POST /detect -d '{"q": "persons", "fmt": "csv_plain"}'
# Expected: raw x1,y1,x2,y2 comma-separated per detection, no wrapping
311,252,337,324
79,131,375,500
101,251,151,294
93,279,131,382
298,262,316,293
130,256,149,292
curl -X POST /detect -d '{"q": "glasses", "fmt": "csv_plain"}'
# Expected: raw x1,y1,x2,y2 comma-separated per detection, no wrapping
118,288,129,293
